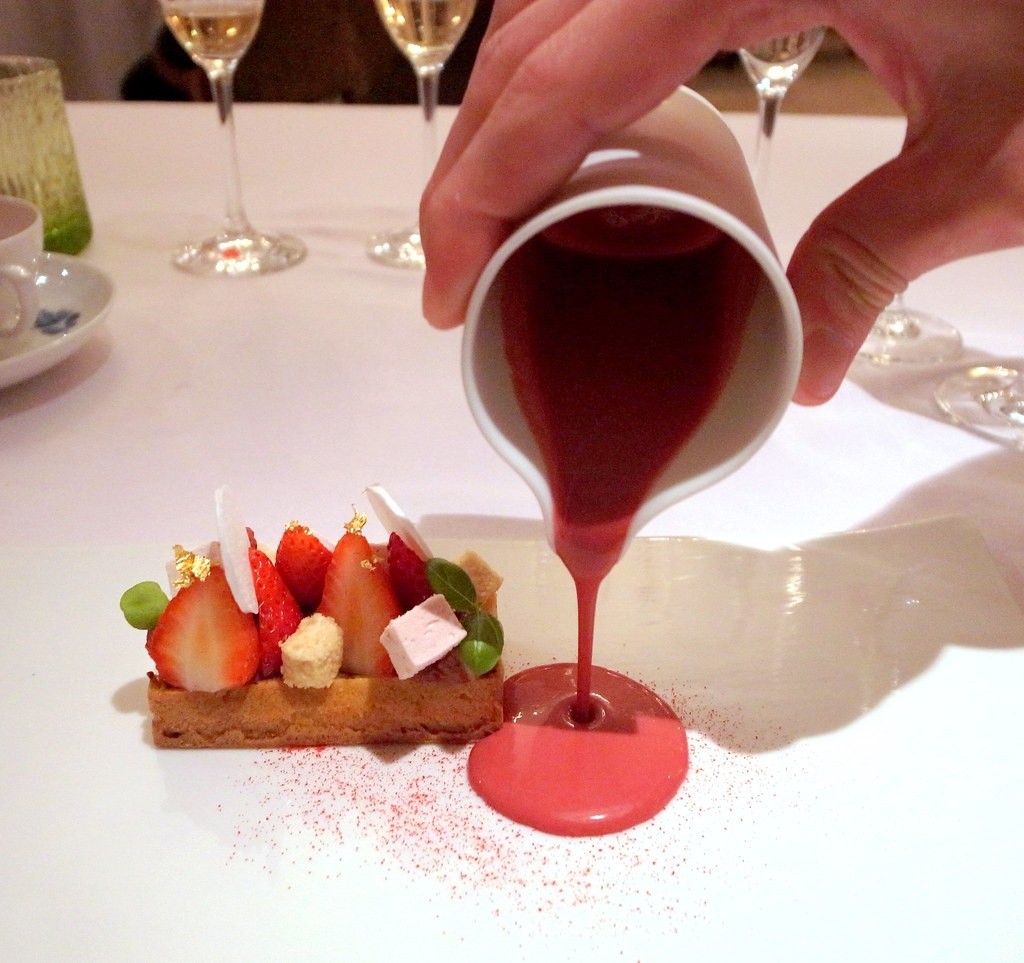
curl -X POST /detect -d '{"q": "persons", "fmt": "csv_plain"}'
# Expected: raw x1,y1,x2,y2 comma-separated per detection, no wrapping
419,0,1024,407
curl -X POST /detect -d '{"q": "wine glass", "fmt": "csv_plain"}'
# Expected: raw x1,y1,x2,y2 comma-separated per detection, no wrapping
935,364,1023,447
159,0,309,277
737,29,827,190
856,289,962,370
368,1,476,268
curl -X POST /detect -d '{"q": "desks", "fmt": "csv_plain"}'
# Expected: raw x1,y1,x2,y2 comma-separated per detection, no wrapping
0,100,1023,963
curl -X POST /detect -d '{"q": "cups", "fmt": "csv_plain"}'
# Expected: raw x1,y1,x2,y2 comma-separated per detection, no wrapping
457,85,803,565
0,195,46,341
0,54,93,257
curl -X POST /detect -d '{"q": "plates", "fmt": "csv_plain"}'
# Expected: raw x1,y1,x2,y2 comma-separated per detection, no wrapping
0,252,117,390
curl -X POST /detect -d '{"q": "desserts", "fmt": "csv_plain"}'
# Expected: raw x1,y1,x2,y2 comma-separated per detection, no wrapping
120,486,502,748
